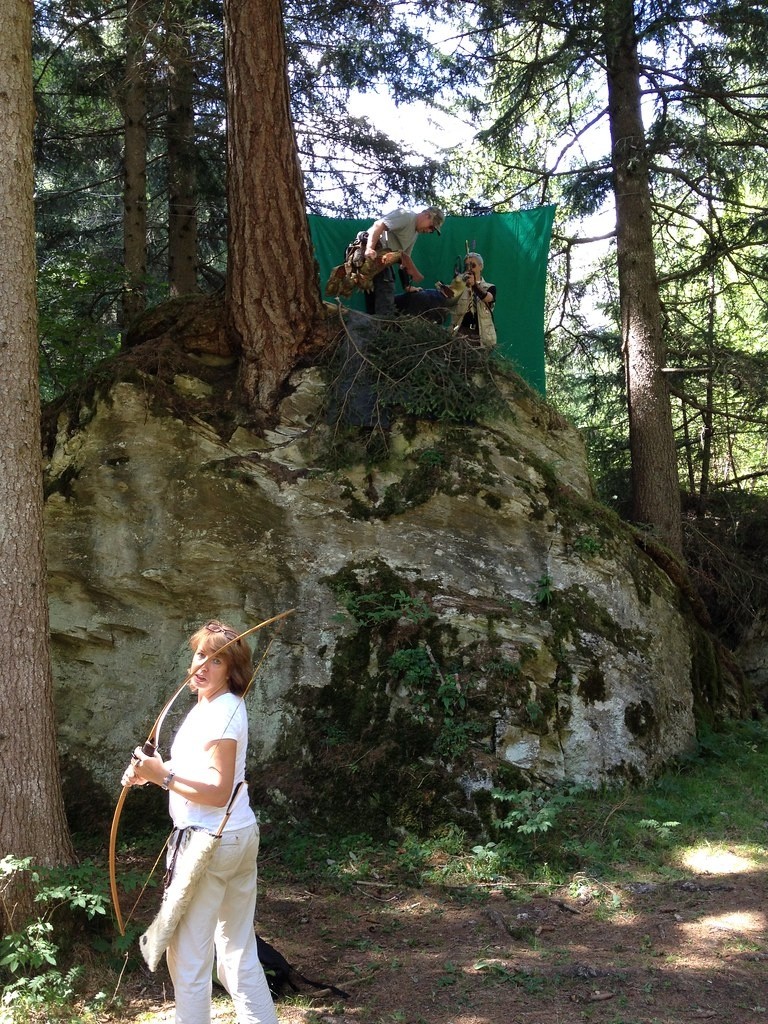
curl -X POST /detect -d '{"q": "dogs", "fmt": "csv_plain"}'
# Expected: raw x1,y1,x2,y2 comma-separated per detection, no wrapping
255,930,350,999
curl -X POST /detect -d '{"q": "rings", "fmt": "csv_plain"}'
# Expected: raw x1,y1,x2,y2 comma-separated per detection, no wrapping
133,759,140,766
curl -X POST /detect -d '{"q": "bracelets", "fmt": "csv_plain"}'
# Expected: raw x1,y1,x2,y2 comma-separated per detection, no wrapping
162,772,176,791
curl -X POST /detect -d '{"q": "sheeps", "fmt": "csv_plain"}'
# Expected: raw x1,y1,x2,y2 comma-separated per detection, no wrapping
394,255,470,324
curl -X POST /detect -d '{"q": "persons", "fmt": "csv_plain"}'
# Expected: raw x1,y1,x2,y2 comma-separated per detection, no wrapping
120,622,277,1024
344,206,498,349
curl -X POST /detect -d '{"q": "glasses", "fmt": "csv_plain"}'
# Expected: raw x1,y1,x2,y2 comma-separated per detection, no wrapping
428,225,434,233
472,262,481,268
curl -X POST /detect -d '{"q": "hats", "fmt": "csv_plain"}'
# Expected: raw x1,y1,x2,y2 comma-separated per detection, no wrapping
427,206,445,236
